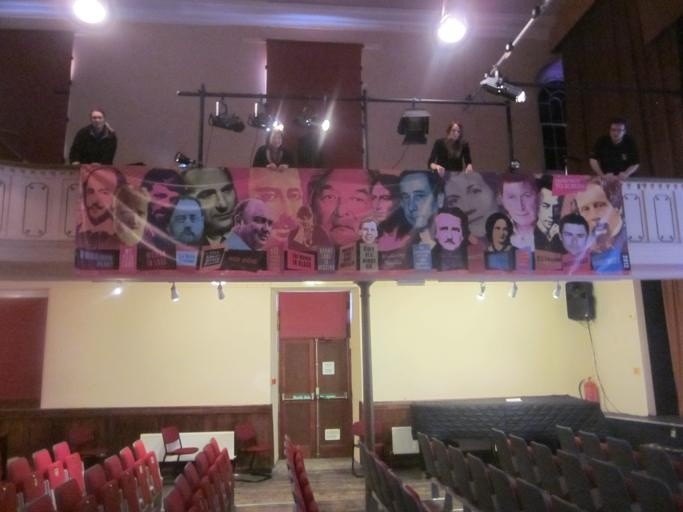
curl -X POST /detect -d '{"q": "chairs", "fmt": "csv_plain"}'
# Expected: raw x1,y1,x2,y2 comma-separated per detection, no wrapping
0,418,683,512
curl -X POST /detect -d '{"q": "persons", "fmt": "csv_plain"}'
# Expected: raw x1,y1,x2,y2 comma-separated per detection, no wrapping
588,116,641,184
252,129,296,171
82,166,628,251
427,119,473,178
68,106,118,166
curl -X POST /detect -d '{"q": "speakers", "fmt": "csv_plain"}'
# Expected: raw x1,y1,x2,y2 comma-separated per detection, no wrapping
565,282,594,320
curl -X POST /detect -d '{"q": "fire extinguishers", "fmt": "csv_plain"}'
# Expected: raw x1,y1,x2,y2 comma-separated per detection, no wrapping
579,377,599,402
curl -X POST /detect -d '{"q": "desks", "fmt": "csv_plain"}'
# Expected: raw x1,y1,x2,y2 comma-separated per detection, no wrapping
409,394,608,440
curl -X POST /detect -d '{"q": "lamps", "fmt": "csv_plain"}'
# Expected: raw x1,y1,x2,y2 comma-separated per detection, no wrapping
217,282,226,299
552,281,562,299
435,0,467,43
476,282,486,301
478,0,558,103
397,102,431,144
171,282,178,302
207,94,330,133
510,282,518,298
